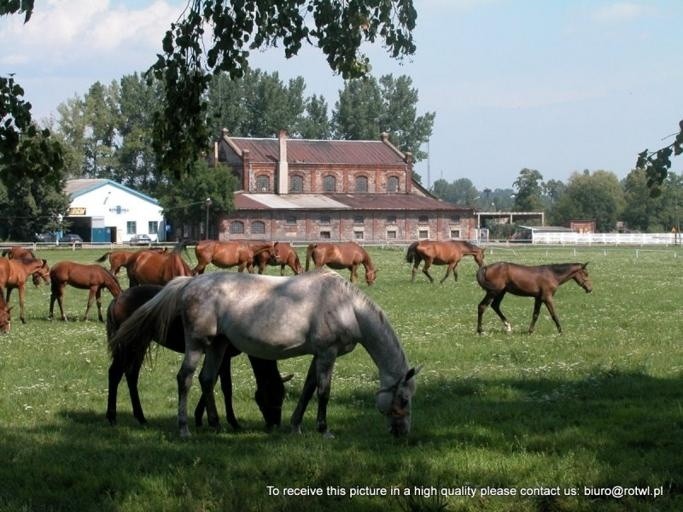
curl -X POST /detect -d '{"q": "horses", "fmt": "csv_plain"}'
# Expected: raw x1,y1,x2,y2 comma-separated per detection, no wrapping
405,239,487,284
1,245,51,333
48,261,124,323
97,236,425,441
475,261,594,337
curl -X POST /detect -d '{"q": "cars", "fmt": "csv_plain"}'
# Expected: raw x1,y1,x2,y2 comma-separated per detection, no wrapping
59,234,83,247
130,233,152,246
